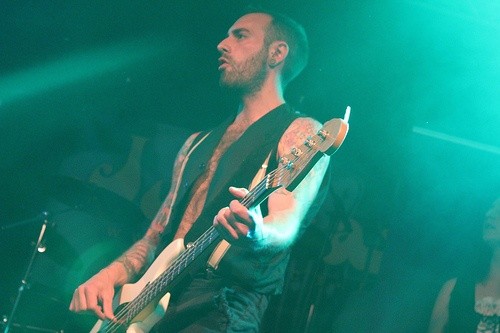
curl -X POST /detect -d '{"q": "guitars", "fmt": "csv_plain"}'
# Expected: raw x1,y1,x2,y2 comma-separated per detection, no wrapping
87,105,352,333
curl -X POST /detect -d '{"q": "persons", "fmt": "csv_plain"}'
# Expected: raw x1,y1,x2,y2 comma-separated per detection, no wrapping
427,201,500,332
69,8,331,333
